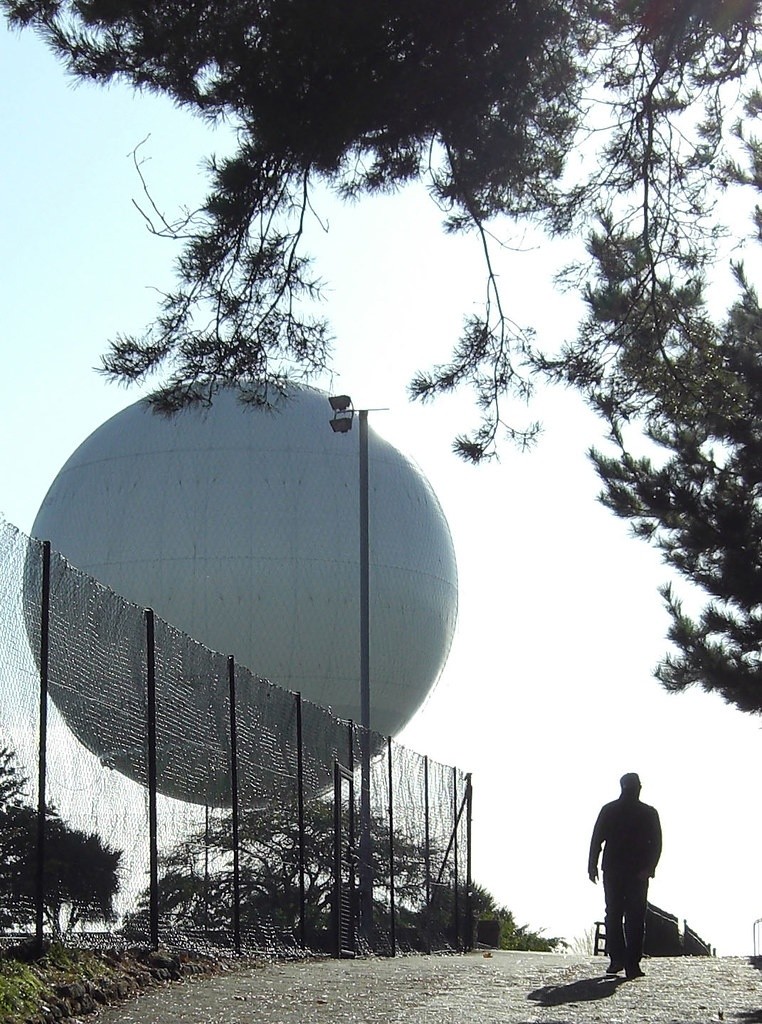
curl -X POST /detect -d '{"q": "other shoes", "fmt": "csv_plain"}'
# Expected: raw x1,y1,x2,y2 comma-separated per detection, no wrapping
626,962,645,977
606,960,623,973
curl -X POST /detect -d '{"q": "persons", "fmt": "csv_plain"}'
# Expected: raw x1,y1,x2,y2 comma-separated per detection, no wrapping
588,773,662,980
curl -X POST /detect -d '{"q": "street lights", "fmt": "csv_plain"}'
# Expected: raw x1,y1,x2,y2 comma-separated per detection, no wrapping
328,394,389,945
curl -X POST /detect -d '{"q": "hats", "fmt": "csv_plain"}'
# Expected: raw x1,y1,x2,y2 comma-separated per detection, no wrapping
620,773,640,789
